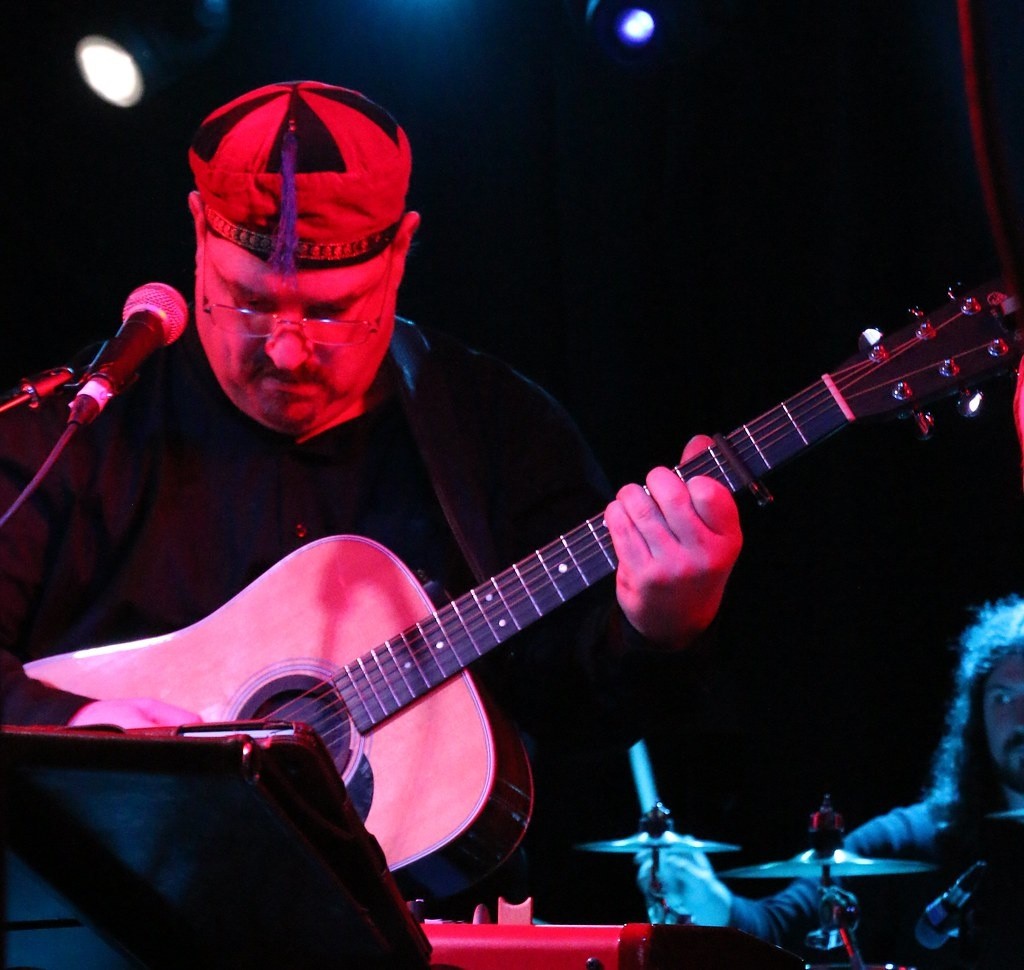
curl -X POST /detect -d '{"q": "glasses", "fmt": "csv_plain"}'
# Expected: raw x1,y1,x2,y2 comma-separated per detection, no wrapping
202,227,397,345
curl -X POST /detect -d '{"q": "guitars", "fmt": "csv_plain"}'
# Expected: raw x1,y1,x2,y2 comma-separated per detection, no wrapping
10,272,1021,910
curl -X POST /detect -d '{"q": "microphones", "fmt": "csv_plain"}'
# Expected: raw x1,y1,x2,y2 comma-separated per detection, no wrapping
913,858,988,951
66,281,188,431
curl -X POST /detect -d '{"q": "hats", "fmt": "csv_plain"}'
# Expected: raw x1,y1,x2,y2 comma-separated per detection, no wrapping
188,81,412,260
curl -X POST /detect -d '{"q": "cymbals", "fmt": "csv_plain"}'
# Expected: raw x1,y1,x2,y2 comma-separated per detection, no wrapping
712,846,939,880
574,828,744,856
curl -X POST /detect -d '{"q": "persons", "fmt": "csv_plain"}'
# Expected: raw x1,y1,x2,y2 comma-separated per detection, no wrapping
0,80,745,920
635,593,1024,969
